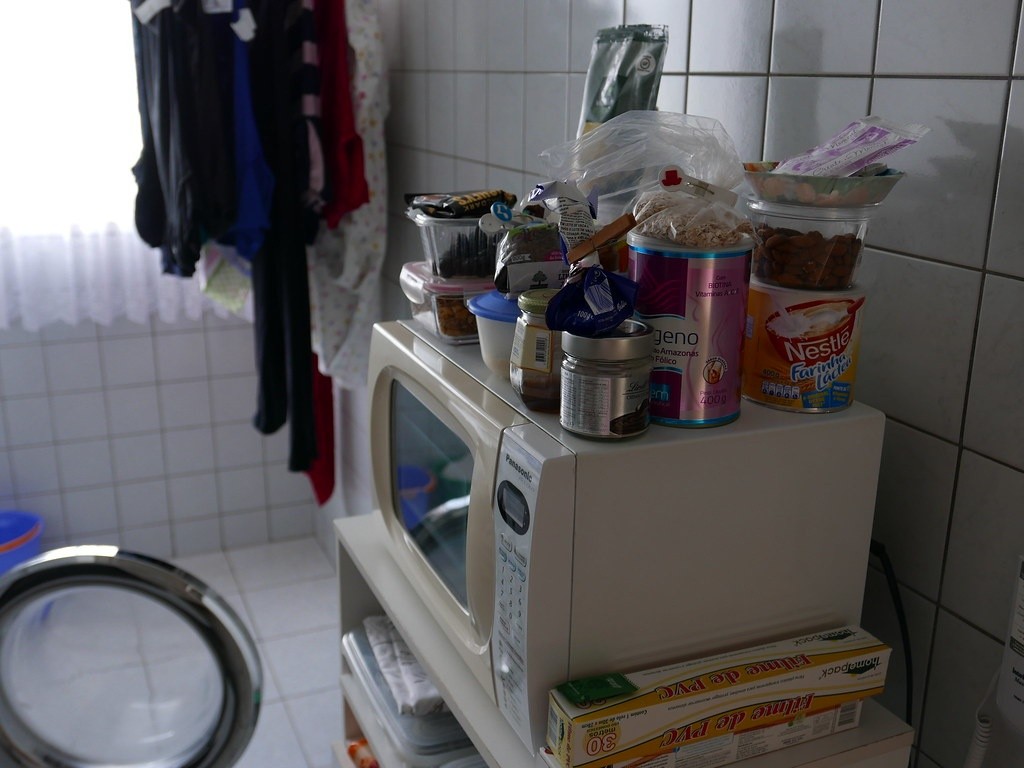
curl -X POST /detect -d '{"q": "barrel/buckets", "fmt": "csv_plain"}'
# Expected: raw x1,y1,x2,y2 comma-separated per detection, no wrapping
509,289,563,412
0,508,46,575
628,230,754,429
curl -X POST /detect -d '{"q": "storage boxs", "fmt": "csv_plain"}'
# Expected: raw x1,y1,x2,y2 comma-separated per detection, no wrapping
539,625,894,768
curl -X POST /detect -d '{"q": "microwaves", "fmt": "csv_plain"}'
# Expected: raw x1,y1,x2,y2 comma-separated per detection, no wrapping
367,319,886,759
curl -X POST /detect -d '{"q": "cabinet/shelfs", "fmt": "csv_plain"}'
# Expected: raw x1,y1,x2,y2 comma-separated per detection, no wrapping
338,514,549,768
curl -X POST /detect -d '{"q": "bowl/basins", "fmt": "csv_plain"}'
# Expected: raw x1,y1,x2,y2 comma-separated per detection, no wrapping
400,261,497,345
466,291,523,380
744,194,882,294
404,205,499,283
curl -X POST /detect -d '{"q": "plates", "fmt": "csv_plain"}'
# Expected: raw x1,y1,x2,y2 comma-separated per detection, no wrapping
742,162,903,207
